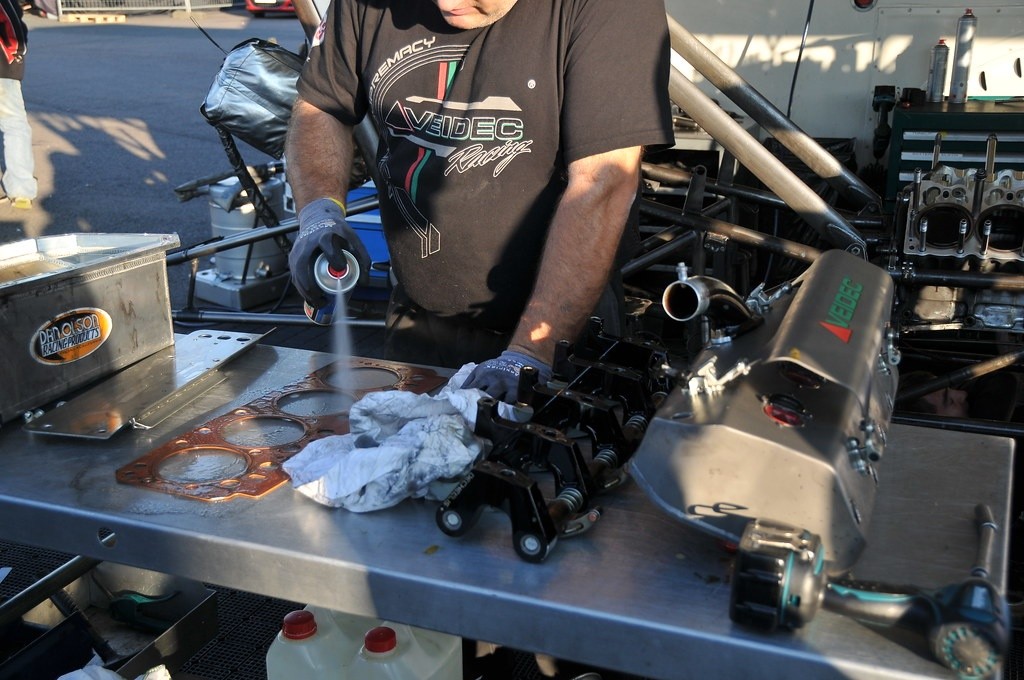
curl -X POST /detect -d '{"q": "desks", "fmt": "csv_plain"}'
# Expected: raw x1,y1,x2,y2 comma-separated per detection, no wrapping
0,332,1017,680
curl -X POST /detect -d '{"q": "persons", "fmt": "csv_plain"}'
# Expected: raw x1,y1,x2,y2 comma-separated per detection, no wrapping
0,0,37,209
285,1,676,408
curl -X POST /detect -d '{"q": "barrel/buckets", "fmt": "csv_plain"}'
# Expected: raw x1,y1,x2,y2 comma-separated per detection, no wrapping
349,618,464,680
263,603,377,680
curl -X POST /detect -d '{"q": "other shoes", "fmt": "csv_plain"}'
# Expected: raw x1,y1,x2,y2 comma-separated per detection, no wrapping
10,195,33,210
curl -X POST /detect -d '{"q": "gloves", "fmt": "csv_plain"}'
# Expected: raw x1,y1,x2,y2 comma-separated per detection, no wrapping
459,350,555,407
288,197,372,311
